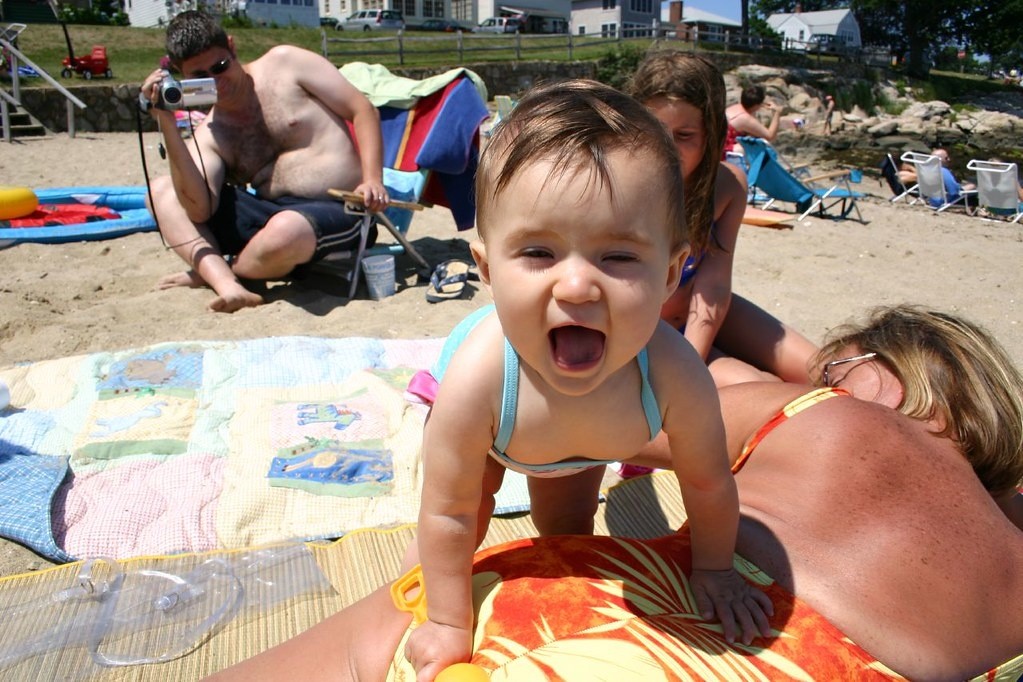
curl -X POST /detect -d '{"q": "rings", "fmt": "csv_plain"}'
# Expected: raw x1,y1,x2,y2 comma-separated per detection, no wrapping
146,83,153,89
378,196,384,200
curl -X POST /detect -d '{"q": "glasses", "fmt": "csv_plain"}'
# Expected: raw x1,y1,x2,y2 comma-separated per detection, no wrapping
824,353,878,386
196,56,234,80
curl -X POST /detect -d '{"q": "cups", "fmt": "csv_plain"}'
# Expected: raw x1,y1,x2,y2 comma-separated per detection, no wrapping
361,255,395,300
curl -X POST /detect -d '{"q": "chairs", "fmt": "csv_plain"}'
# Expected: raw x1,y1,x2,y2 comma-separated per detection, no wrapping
880,151,1023,223
726,137,870,226
321,62,518,299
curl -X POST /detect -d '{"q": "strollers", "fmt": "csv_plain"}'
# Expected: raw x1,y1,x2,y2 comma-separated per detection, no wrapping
0,23,27,71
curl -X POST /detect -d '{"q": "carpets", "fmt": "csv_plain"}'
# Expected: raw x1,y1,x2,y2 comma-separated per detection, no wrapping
0,336,670,564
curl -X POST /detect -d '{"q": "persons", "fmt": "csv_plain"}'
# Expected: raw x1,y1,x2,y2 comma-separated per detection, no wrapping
202,45,1023,682
141,12,391,313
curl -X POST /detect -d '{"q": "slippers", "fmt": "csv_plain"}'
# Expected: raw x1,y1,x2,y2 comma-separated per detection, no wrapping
424,257,470,303
419,267,476,282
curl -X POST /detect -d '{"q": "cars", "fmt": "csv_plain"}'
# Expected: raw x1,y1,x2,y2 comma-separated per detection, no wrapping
805,34,846,56
471,17,521,35
335,9,407,34
420,18,472,33
60,45,113,80
320,17,338,27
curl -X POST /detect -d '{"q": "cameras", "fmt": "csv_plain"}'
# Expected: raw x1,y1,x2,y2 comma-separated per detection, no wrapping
156,68,218,109
